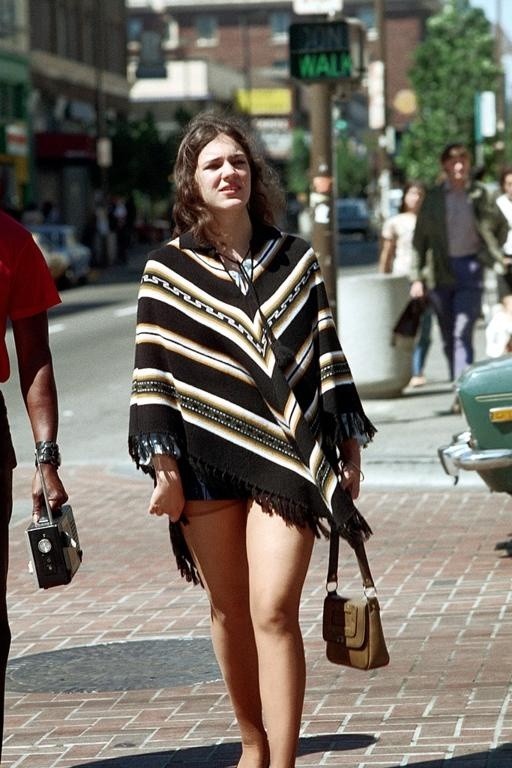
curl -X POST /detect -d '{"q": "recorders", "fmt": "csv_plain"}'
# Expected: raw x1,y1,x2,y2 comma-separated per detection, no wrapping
24,503,84,590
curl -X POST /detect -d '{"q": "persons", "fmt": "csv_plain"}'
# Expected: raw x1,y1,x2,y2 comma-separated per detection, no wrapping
380,181,434,387
409,140,512,383
493,168,512,314
1,209,72,758
125,112,379,768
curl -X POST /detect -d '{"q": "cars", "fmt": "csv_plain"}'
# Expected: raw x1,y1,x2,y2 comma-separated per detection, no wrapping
336,197,369,238
434,349,512,500
30,223,92,286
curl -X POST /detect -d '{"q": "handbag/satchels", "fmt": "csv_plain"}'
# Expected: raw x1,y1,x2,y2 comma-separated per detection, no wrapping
320,477,392,672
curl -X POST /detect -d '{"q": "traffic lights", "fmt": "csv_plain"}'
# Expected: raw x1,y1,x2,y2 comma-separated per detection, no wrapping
286,16,368,85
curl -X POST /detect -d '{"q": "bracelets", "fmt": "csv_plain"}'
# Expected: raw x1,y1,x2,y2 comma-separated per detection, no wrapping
32,439,62,469
349,461,365,481
